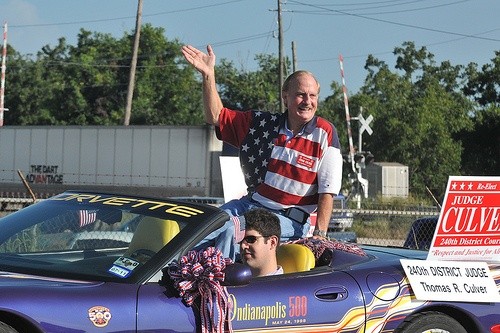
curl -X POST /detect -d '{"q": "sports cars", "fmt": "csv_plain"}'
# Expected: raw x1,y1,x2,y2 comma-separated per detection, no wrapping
0,189,500,333
78,197,357,249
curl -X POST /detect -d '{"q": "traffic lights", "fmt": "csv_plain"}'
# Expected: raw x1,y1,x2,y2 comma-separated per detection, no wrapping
355,151,375,166
352,120,361,139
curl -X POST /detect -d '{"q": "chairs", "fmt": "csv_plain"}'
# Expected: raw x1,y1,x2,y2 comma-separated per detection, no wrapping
122,216,179,265
275,244,315,274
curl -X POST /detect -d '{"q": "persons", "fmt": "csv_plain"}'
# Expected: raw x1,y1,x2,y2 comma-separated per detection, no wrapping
180,43,343,259
239,210,284,277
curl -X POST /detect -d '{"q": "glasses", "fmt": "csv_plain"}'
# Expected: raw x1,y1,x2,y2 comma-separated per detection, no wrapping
237,236,272,243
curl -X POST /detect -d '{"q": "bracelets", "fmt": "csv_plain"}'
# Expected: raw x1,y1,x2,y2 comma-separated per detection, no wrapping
312,229,327,238
